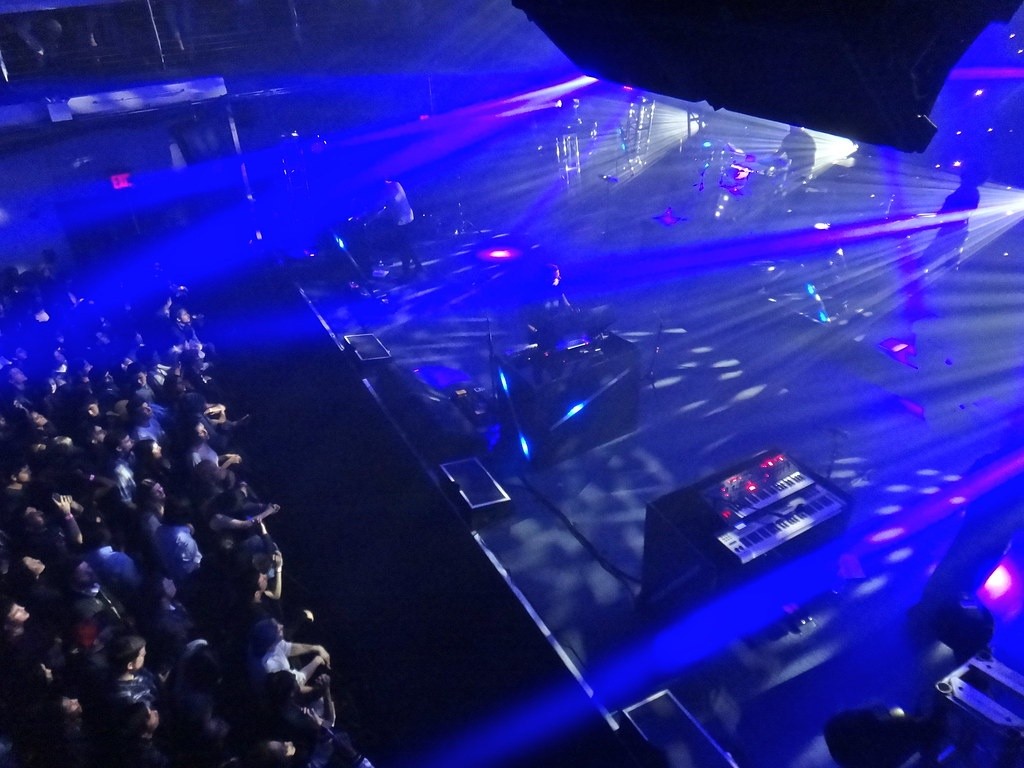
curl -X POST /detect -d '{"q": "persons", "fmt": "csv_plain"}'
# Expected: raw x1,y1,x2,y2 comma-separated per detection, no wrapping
384,176,422,279
771,126,816,192
0,250,371,768
526,264,578,386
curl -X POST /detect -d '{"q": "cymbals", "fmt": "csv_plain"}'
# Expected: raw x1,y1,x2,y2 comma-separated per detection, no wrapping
757,156,788,165
726,142,745,157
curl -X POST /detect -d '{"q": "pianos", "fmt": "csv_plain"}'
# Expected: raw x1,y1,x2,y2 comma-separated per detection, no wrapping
640,444,856,634
490,300,641,471
